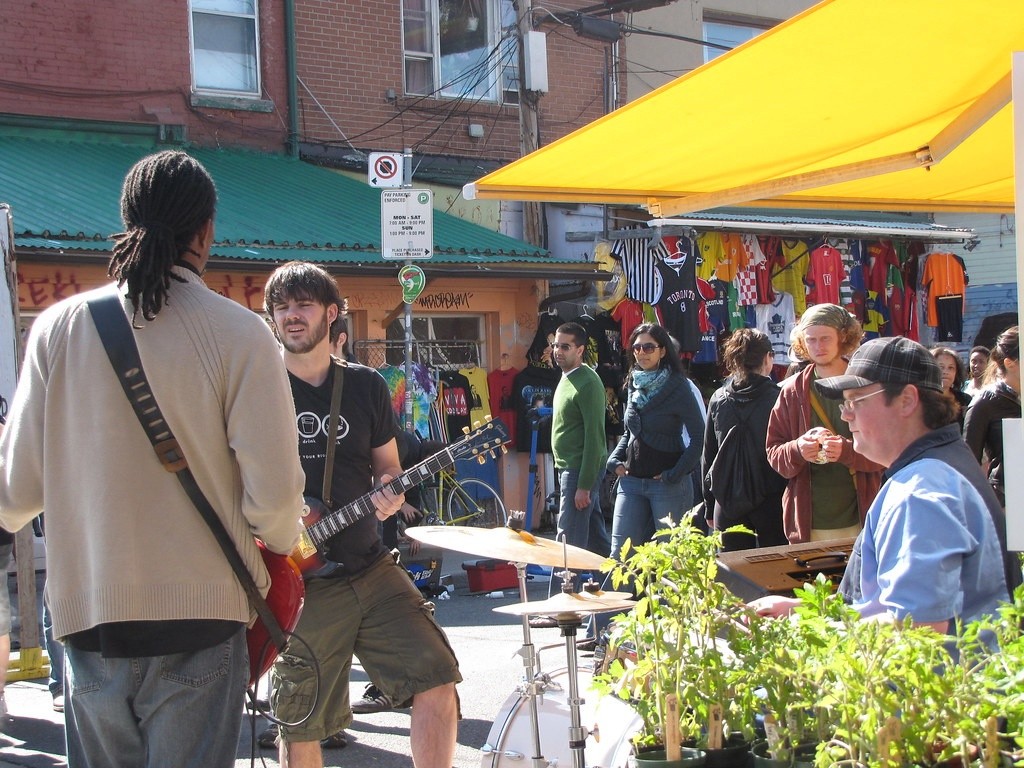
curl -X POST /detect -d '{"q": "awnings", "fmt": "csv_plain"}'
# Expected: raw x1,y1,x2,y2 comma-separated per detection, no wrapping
464,0,1024,217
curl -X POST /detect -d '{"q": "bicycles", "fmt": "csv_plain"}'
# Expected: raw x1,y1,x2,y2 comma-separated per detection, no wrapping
419,441,507,531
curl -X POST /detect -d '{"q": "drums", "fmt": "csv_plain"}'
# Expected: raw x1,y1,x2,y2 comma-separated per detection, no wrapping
478,663,647,768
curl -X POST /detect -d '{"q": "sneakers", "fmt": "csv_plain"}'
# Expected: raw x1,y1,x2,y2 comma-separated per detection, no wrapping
256,723,281,748
53,690,64,712
351,679,414,713
247,698,271,711
319,728,346,749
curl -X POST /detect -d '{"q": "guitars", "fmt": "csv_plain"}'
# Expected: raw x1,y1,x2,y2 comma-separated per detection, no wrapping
246,538,306,691
288,413,514,576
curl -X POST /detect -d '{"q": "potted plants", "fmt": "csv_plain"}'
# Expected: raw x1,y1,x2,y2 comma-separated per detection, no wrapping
596,502,1024,767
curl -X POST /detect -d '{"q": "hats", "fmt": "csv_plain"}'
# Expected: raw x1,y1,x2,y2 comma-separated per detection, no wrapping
814,334,944,401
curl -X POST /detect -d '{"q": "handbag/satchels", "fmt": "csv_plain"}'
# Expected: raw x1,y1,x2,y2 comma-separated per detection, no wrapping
609,474,620,506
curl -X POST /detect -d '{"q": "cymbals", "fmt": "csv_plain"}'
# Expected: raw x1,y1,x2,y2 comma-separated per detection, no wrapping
579,590,634,600
404,525,607,570
491,593,637,620
805,426,834,465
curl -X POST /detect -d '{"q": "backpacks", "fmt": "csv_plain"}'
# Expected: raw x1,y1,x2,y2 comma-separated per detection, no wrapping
707,388,765,520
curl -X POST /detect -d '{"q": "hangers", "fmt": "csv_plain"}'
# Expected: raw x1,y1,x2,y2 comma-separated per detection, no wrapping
581,304,594,320
664,241,702,265
887,283,893,289
819,234,832,248
939,285,962,299
549,302,560,317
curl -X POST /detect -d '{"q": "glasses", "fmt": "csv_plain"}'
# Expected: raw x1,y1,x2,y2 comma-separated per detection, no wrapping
632,343,661,354
552,343,582,350
839,388,886,413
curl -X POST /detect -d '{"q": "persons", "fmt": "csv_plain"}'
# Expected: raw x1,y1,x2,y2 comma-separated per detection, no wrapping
929,347,973,434
767,302,887,544
963,325,1022,512
264,261,464,768
0,151,310,768
576,323,706,652
702,335,1024,735
529,322,611,628
702,328,789,552
962,346,990,397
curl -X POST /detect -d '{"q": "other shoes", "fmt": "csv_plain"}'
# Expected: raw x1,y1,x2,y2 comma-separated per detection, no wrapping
528,613,582,627
576,640,604,651
